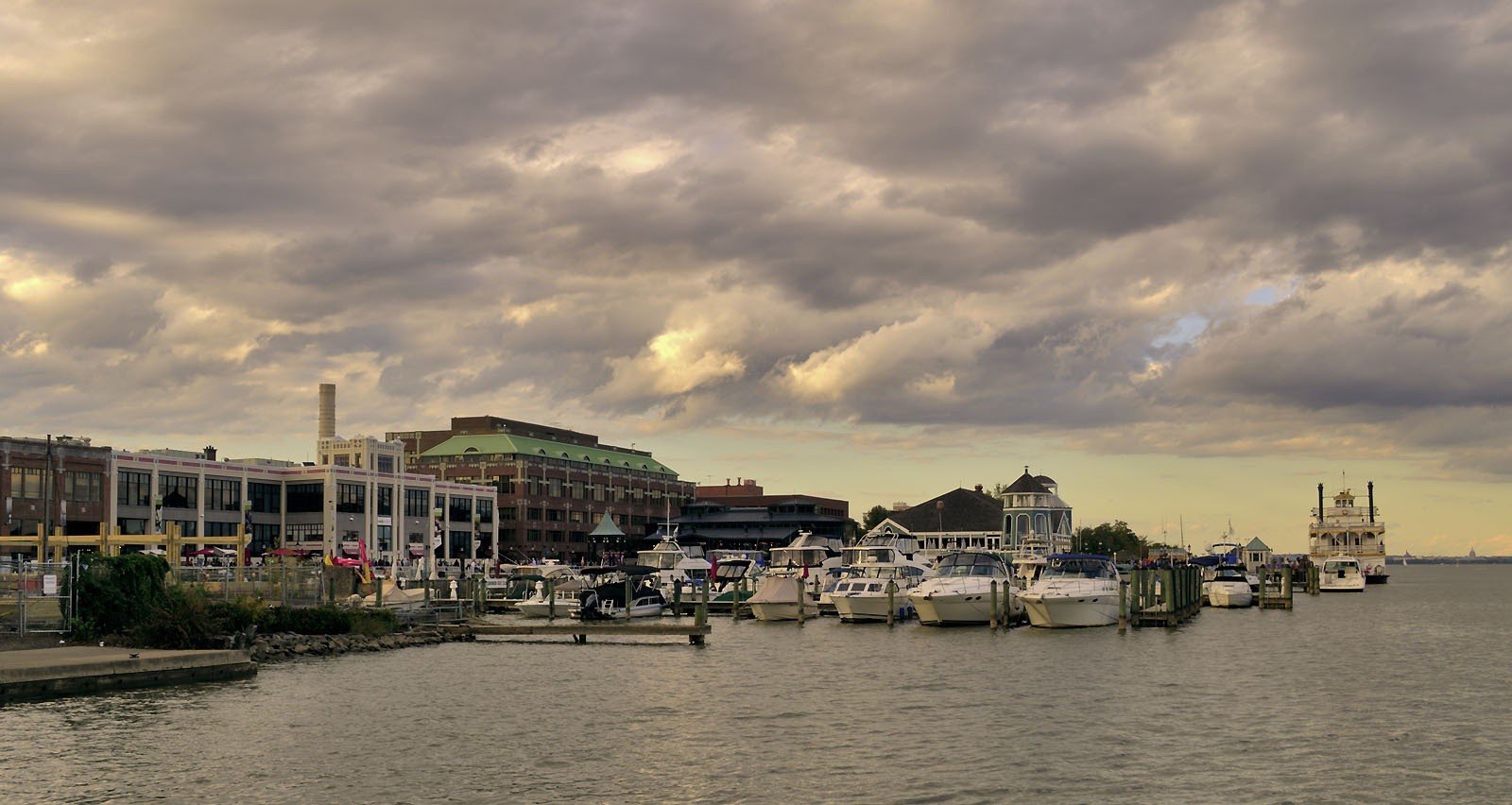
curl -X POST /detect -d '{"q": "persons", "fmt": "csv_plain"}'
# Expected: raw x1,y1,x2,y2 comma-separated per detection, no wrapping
1119,558,1187,570
1265,555,1312,581
189,558,235,568
371,551,625,576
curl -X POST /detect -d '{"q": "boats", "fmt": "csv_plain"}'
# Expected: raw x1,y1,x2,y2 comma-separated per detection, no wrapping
636,529,1020,599
1016,555,1138,629
599,594,662,618
1319,554,1366,592
660,558,763,611
908,545,1026,628
831,556,935,625
1208,566,1254,607
747,575,824,621
514,580,579,620
1306,479,1389,584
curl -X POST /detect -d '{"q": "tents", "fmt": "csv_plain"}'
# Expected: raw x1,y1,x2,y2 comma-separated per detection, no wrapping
332,556,363,568
187,547,237,566
263,549,306,557
